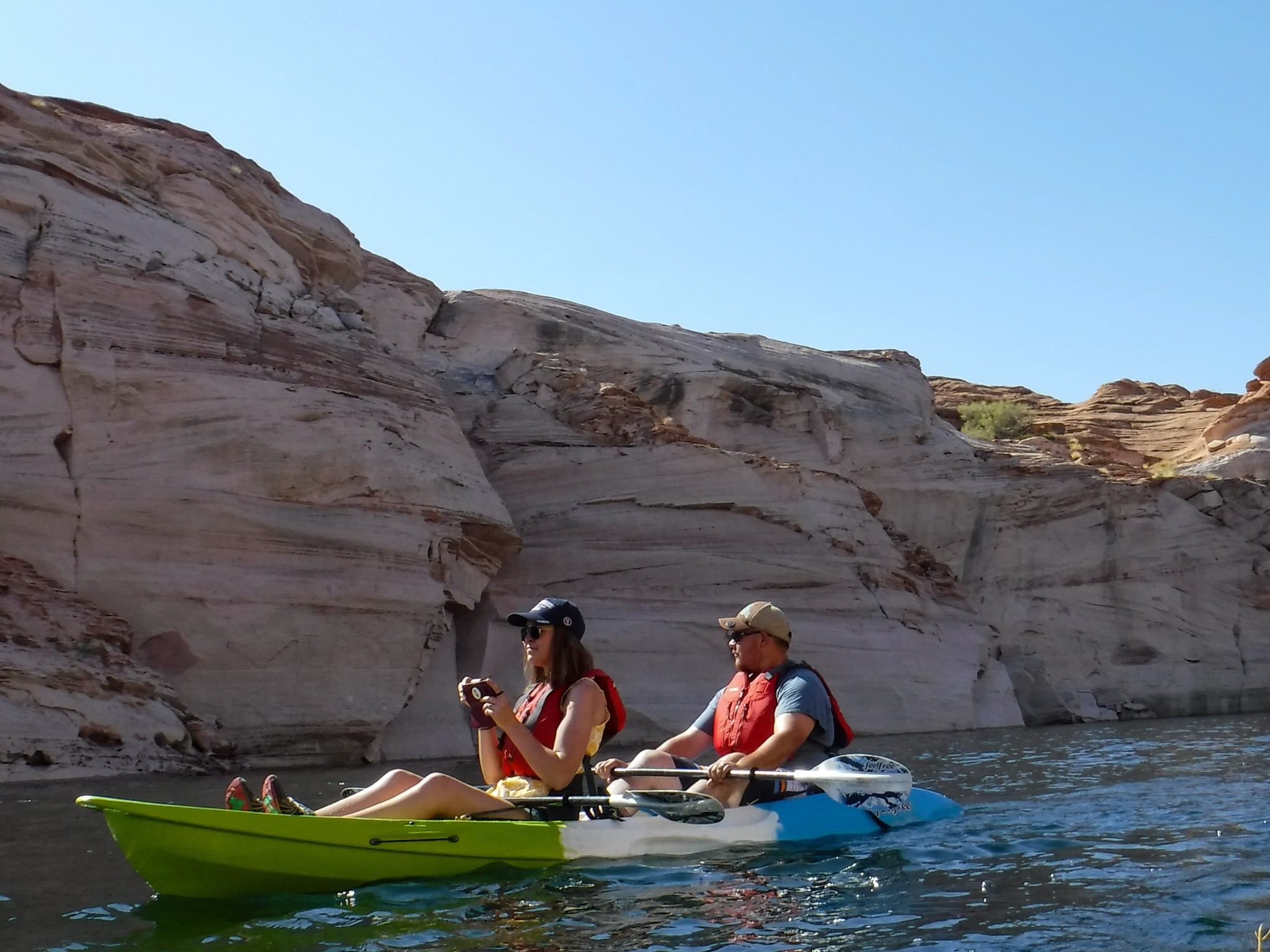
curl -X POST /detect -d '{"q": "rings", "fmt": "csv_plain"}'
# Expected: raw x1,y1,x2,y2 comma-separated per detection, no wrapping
496,690,504,696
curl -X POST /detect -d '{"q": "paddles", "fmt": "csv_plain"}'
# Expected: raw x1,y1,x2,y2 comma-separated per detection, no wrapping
590,754,913,811
341,787,725,825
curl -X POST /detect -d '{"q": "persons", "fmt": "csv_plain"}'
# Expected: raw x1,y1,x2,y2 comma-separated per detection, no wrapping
581,601,840,821
224,597,611,820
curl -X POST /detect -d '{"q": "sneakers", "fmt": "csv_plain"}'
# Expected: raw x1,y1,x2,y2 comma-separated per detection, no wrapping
261,774,316,816
225,777,265,812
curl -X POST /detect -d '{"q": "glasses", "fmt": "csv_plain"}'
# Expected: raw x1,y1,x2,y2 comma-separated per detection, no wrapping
725,630,772,642
521,625,555,641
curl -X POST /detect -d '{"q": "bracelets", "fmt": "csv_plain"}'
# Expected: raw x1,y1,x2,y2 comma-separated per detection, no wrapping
478,726,495,730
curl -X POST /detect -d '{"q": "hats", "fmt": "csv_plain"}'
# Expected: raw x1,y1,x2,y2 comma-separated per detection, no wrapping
507,597,585,641
718,601,792,644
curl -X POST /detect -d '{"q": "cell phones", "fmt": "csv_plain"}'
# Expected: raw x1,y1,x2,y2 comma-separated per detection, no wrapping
460,680,496,730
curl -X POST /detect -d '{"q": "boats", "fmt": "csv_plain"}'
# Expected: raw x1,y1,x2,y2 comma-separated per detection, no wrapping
74,790,964,894
